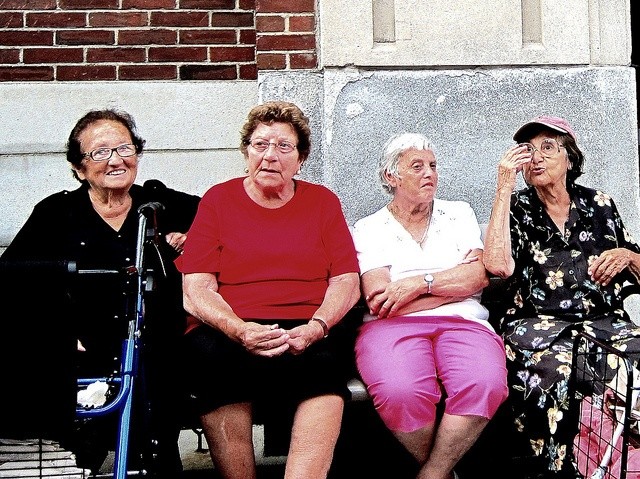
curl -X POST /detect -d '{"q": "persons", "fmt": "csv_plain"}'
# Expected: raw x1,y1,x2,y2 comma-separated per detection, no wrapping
352,131,508,478
173,101,360,479
0,106,202,479
481,117,640,479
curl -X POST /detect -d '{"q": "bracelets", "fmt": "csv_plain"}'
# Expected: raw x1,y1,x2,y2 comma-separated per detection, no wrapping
308,317,328,338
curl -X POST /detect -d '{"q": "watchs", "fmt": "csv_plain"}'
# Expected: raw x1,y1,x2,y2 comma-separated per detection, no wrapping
423,273,434,295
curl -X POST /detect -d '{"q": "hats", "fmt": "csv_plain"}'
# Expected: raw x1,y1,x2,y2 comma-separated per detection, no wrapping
513,115,575,143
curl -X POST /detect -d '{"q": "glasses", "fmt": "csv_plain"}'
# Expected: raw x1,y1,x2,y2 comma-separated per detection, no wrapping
518,138,565,157
249,137,297,152
83,143,138,161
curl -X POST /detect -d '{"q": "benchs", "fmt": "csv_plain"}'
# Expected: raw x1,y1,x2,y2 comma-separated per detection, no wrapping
345,276,639,404
